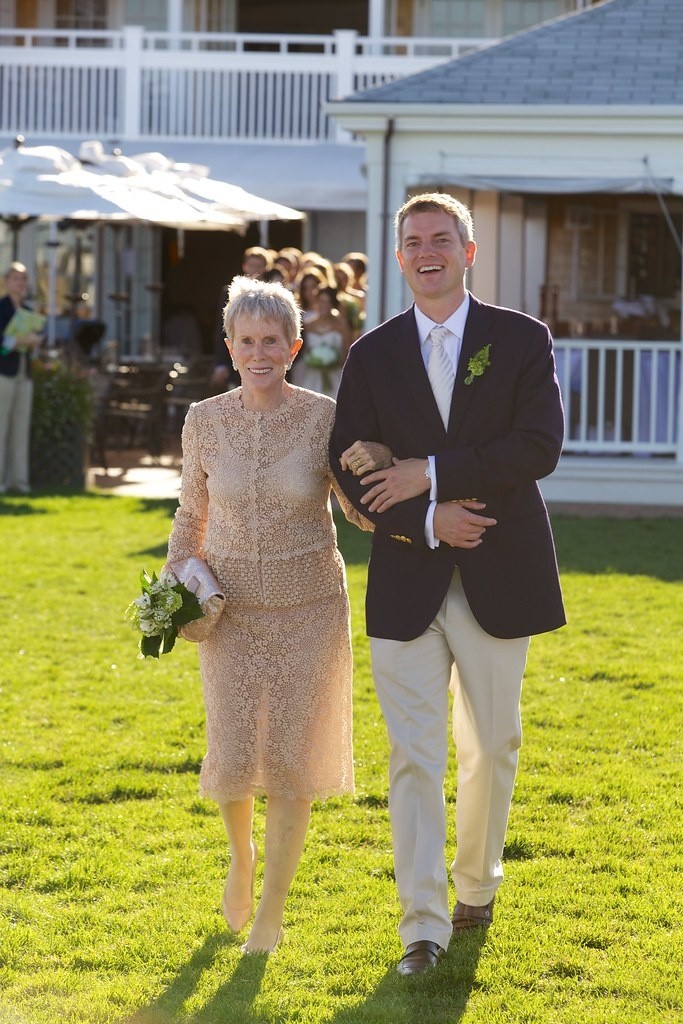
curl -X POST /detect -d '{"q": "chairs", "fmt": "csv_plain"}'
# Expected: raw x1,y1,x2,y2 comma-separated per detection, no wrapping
71,338,217,471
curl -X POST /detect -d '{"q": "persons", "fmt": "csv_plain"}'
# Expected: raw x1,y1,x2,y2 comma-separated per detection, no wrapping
1,260,46,495
329,190,568,979
209,245,368,397
161,275,395,954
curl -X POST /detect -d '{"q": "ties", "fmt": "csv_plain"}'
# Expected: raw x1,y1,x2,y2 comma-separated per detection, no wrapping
428,327,454,436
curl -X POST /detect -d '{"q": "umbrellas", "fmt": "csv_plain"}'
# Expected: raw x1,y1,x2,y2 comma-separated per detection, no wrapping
0,141,305,370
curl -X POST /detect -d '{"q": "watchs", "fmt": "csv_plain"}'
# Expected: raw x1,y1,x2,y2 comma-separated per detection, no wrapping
425,460,431,489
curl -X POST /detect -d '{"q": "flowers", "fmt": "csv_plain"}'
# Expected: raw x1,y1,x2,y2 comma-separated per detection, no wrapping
305,342,344,393
123,567,206,660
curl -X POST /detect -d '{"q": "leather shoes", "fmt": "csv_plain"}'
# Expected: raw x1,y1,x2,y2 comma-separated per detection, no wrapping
451,897,494,930
222,842,255,936
397,940,439,977
241,927,283,956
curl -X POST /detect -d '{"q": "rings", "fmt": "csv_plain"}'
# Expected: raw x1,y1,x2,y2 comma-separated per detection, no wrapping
351,460,362,469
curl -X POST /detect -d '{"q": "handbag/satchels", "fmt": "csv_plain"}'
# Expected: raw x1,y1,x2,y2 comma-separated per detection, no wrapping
170,556,227,644
